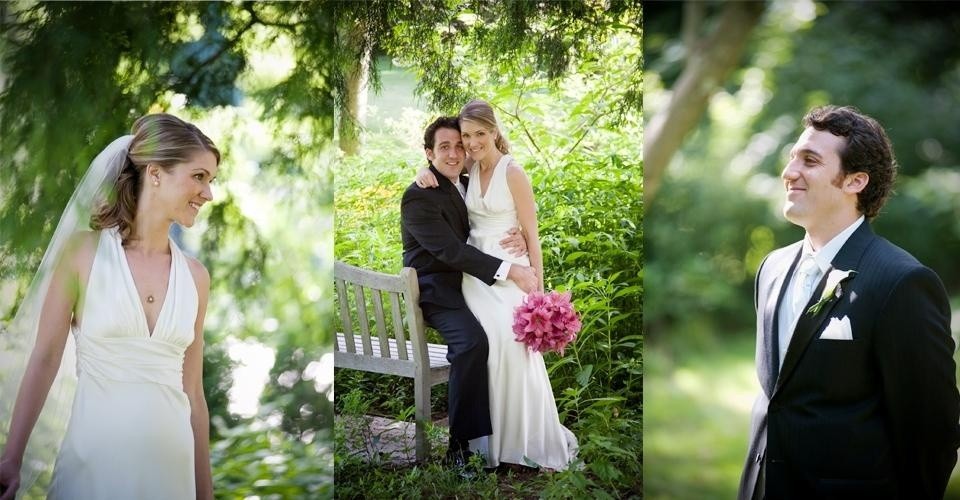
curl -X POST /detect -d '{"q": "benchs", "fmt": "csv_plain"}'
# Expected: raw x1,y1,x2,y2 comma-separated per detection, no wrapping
334,260,454,471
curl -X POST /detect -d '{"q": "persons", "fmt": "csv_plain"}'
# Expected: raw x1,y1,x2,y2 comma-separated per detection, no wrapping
414,98,588,473
0,112,221,500
735,108,958,499
400,116,538,482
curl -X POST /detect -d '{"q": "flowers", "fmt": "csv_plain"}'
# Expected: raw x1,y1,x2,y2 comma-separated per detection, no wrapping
805,270,858,319
511,286,582,358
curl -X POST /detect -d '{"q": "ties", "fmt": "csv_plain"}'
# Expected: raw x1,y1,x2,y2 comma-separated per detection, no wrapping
786,258,825,325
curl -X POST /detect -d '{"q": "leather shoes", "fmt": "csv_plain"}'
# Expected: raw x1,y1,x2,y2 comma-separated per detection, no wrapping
441,451,541,475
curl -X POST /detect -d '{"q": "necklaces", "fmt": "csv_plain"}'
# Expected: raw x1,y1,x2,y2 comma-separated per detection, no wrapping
126,247,166,303
